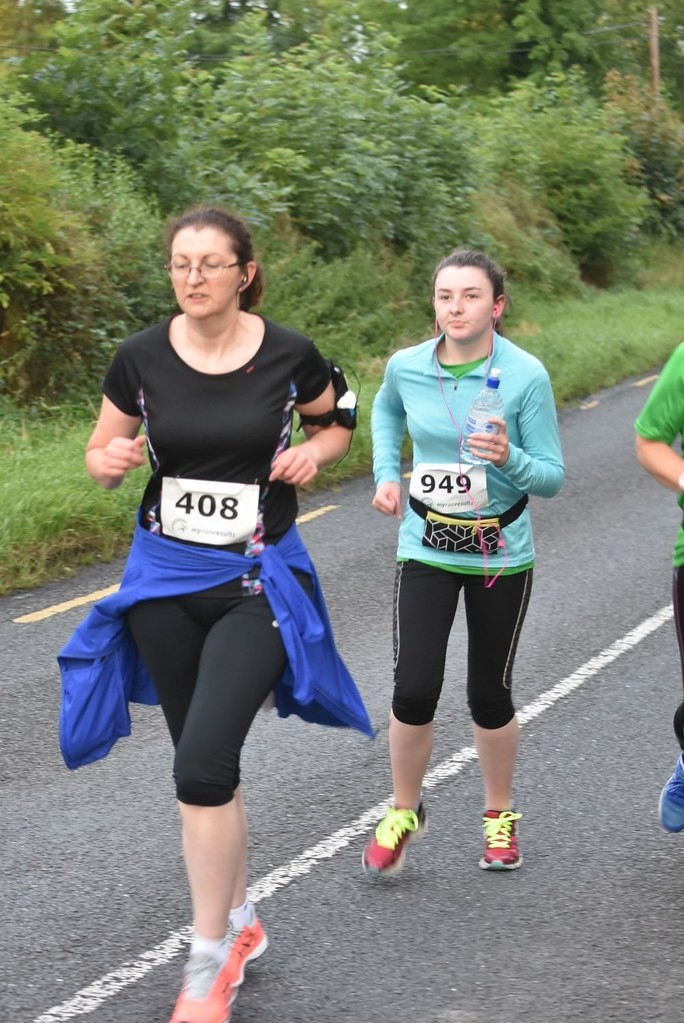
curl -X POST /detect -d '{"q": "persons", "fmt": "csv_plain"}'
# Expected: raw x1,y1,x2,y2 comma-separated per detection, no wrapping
633,340,684,832
362,250,565,872
57,210,371,1023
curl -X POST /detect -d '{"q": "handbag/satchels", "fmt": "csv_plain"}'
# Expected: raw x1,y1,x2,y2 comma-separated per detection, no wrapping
421,510,501,557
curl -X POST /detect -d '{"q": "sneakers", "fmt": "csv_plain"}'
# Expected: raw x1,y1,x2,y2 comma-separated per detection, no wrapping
363,805,426,878
657,751,684,834
478,803,523,870
169,939,238,1023
228,903,268,988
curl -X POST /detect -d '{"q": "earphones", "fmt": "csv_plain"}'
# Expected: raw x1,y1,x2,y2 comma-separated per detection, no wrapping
492,304,499,314
239,273,248,287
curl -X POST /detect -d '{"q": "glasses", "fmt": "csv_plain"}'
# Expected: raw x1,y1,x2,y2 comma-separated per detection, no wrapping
164,253,247,279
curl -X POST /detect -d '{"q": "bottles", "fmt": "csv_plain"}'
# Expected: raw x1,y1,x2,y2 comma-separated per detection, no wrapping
461,368,504,466
